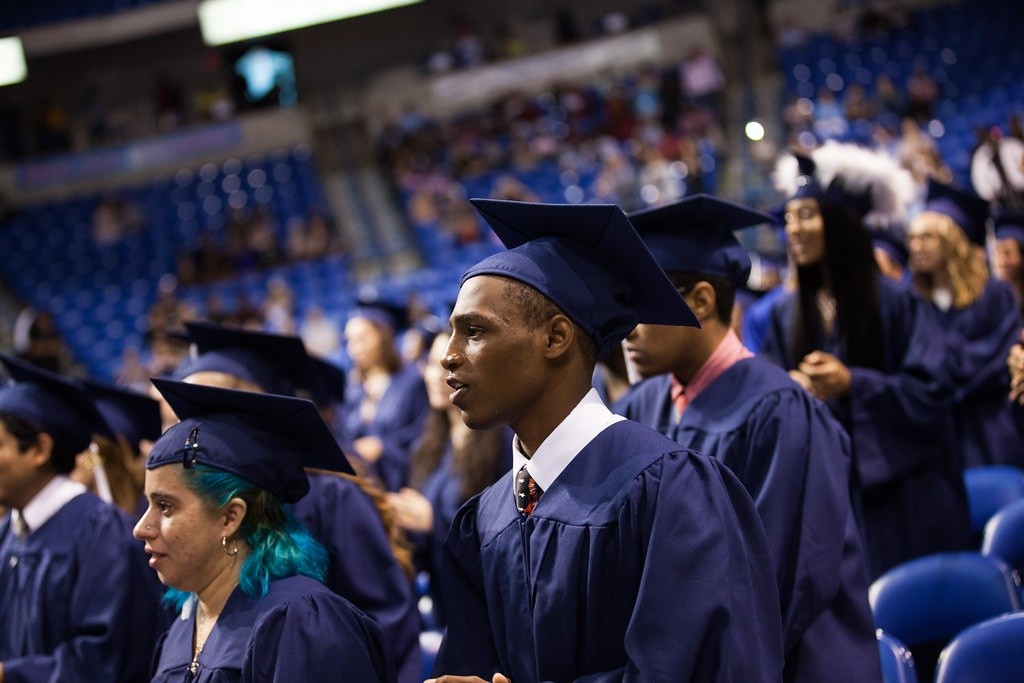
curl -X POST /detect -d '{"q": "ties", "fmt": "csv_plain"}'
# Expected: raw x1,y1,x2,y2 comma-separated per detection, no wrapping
517,467,541,519
673,393,685,426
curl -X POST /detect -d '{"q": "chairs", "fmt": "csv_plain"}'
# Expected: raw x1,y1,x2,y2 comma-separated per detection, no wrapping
867,465,1024,683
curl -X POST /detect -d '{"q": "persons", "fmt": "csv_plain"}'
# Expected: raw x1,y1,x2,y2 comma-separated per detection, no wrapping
0,0,1024,683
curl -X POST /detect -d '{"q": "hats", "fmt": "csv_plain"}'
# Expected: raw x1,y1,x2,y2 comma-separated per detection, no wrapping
0,353,118,450
76,377,162,456
146,376,358,505
344,296,413,341
298,352,346,402
787,146,876,221
628,193,781,285
459,197,702,353
923,177,990,245
181,320,318,398
